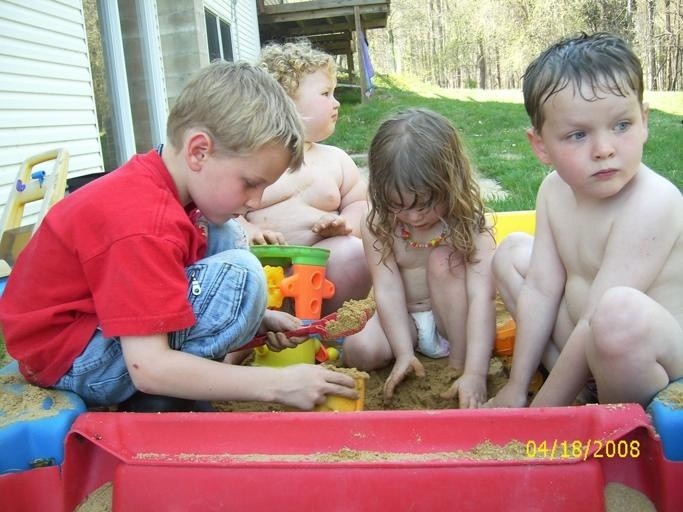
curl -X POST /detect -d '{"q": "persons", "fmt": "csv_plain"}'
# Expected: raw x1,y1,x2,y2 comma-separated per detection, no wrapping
487,32,681,408
0,58,358,410
341,109,495,411
220,40,372,367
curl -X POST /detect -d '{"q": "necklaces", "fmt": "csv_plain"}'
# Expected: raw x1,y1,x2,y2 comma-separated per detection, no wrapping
396,219,451,248
